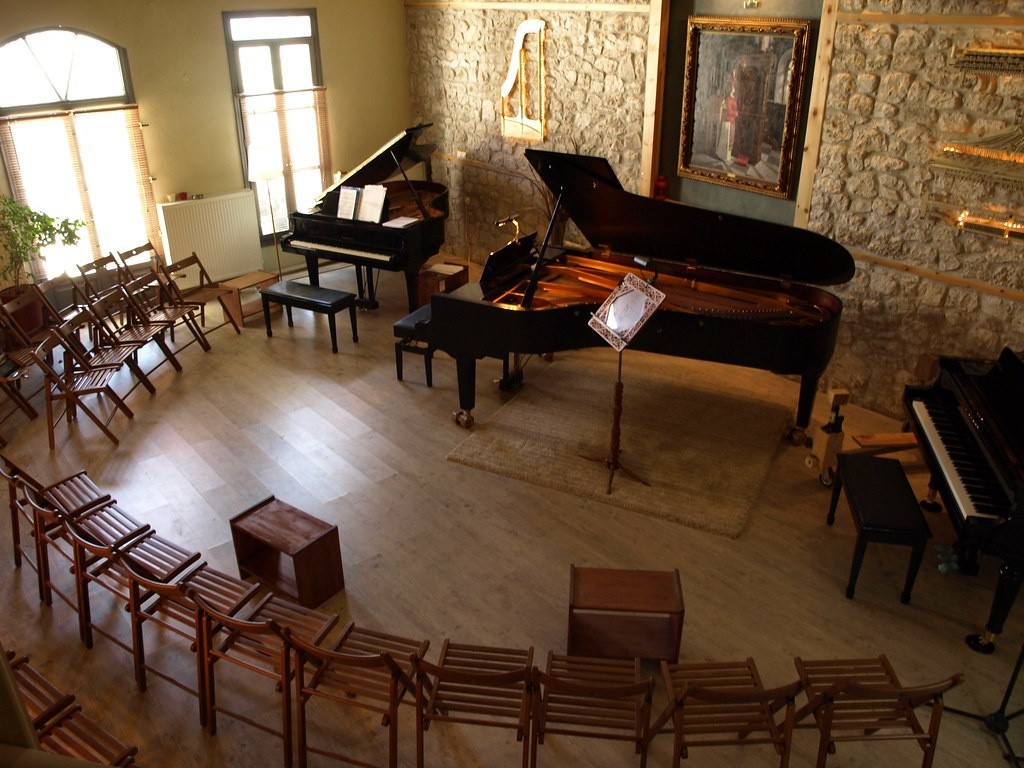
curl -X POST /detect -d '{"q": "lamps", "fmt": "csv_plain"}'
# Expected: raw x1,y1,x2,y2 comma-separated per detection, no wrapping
247,142,291,279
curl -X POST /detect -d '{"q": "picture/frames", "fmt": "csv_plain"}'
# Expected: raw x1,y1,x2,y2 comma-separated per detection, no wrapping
677,15,810,198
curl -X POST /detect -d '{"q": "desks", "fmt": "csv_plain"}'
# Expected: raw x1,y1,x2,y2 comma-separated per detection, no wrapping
566,564,686,660
217,269,282,328
227,497,346,605
419,262,470,302
156,190,264,291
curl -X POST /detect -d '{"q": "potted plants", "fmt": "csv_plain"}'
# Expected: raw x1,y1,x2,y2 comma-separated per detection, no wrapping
0,193,83,336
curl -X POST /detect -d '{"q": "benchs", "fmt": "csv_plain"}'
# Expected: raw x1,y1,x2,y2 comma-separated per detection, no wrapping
825,453,933,605
259,281,358,353
392,304,439,388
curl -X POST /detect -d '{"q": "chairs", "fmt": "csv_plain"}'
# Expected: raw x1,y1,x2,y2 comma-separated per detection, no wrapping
0,238,968,768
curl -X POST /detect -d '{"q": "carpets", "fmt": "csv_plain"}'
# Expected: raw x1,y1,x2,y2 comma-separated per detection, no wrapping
446,354,793,537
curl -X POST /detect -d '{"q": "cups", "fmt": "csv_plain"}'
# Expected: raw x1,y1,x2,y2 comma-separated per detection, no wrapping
193,194,203,200
167,194,175,202
179,192,187,200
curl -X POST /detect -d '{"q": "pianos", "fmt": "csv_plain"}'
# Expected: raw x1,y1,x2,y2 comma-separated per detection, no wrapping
429,147,855,447
279,122,450,314
900,345,1024,655
